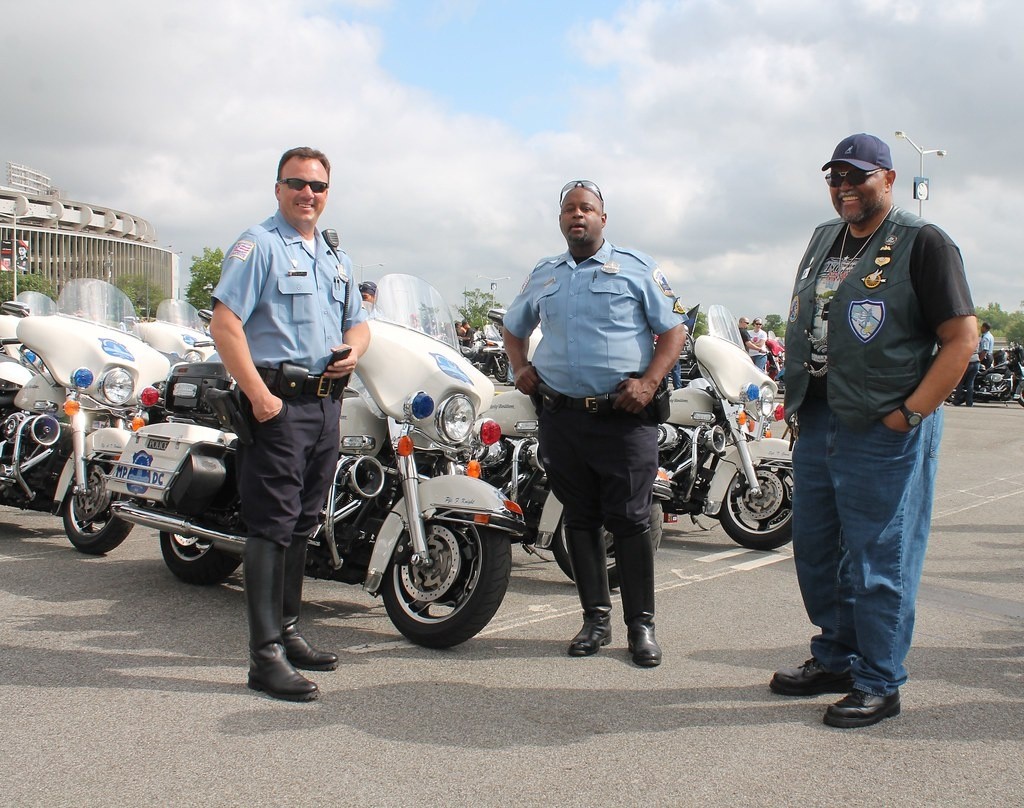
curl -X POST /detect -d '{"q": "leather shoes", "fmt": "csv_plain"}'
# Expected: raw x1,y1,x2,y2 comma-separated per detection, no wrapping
824,683,901,729
768,656,849,695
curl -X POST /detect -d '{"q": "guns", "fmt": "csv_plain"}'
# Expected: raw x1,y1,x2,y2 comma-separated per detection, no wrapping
221,394,238,415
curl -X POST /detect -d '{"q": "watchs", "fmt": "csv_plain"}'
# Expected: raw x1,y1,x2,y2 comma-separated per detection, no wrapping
900,404,923,428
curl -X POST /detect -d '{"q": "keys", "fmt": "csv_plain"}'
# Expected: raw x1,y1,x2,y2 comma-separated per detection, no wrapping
781,413,799,451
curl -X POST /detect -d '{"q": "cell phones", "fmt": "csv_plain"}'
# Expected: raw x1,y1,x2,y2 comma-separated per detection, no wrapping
319,347,352,377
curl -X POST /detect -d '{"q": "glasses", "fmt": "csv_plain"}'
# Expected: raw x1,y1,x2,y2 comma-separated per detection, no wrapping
358,283,373,291
743,321,749,325
559,180,605,212
279,179,330,192
755,323,763,326
825,168,885,188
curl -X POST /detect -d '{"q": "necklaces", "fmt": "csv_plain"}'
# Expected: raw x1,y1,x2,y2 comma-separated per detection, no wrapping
831,205,894,291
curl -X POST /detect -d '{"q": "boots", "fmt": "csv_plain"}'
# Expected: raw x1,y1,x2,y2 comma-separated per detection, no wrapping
614,528,663,664
563,529,610,656
242,539,320,702
280,536,339,671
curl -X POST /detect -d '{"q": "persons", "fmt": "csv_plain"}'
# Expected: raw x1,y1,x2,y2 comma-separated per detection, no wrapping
207,146,371,702
504,363,515,386
738,316,769,370
406,313,424,332
947,346,980,407
358,280,383,319
664,359,682,392
501,180,690,667
454,321,487,349
17,246,28,269
978,321,994,369
766,134,978,728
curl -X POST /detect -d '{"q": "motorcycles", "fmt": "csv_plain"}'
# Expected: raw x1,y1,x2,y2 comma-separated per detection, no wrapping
944,340,1024,409
96,272,527,650
0,278,801,593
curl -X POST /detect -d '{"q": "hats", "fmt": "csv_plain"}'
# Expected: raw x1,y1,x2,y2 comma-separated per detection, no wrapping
822,133,893,172
360,281,377,294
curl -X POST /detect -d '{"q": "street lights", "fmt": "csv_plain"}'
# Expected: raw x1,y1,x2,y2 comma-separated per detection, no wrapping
894,131,946,219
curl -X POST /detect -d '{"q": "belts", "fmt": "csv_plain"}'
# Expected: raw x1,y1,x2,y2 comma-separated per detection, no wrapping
538,384,614,414
262,368,333,397
